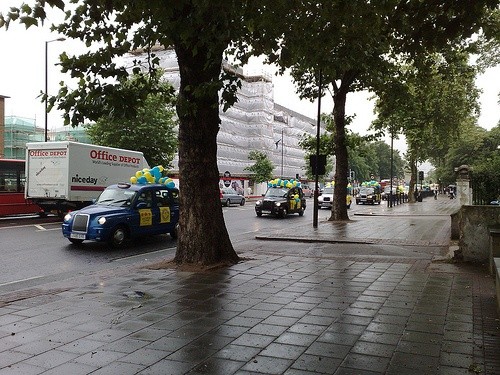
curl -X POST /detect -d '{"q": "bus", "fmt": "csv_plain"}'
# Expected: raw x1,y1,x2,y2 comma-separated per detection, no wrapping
0,159,48,217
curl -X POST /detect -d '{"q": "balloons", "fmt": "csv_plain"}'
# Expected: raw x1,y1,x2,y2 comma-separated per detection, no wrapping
130,164,174,188
361,181,381,187
327,180,353,206
268,177,302,188
294,194,300,202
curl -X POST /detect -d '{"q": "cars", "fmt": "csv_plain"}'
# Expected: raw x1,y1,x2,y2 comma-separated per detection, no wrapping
356,184,427,205
220,188,245,207
255,186,306,218
61,183,181,251
300,186,350,209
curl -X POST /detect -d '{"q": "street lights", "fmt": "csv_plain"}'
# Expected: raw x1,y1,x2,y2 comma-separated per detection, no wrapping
281,126,294,175
45,38,66,142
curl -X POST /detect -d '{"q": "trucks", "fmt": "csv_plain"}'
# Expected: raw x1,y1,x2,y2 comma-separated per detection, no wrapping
24,141,151,221
